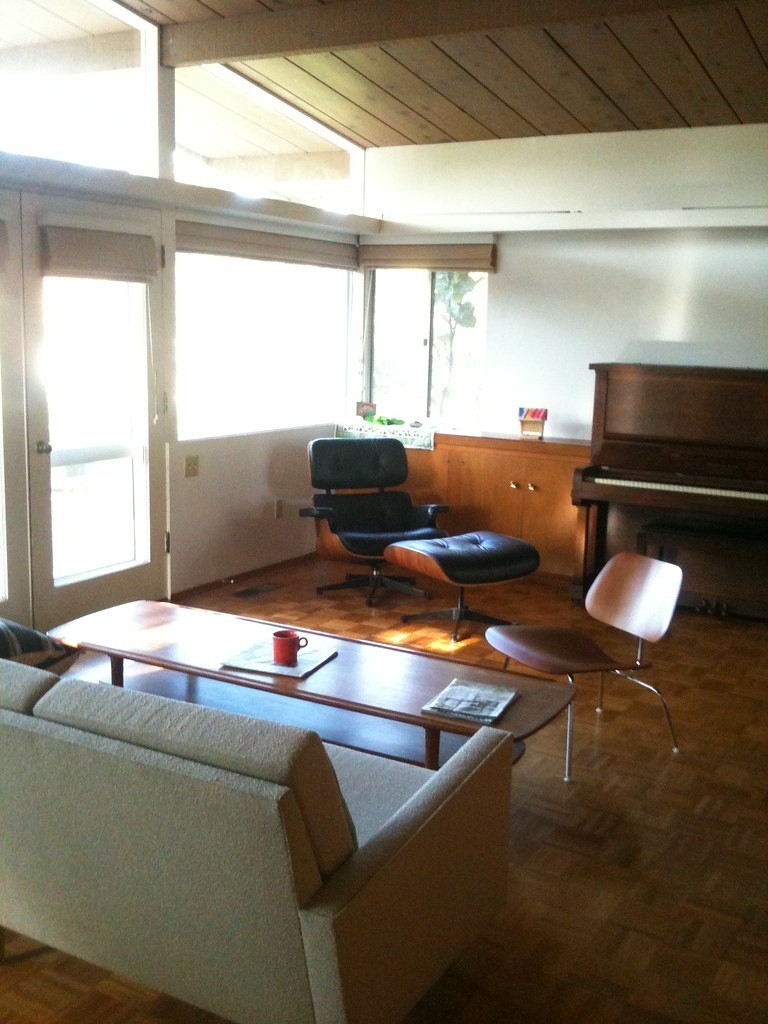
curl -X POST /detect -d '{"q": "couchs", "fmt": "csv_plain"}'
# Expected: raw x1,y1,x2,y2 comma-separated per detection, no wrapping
1,659,514,1024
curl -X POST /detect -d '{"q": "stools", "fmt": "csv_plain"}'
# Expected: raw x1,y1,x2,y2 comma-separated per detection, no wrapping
383,529,541,644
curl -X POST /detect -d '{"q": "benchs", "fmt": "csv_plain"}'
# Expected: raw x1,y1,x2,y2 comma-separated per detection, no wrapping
636,515,767,623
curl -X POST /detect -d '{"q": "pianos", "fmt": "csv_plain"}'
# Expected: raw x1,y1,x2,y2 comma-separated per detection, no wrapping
571,360,768,604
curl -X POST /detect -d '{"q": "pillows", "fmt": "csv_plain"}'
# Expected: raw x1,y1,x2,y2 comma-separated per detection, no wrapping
0,615,68,670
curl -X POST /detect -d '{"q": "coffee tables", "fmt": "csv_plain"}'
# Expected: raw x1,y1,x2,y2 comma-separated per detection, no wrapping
44,599,579,771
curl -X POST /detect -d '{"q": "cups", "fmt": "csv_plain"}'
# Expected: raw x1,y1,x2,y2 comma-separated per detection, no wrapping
273,630,307,666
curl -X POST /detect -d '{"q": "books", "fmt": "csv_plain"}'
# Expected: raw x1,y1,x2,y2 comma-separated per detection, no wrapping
420,678,519,725
219,637,338,678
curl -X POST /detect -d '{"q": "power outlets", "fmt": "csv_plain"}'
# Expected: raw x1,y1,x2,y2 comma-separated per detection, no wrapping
275,499,283,518
184,455,199,477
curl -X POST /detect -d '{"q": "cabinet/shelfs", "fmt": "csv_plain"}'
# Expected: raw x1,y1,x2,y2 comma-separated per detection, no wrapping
444,449,593,580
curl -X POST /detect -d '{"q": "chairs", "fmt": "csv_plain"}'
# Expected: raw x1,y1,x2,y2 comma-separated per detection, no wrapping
298,436,450,607
486,550,684,782
